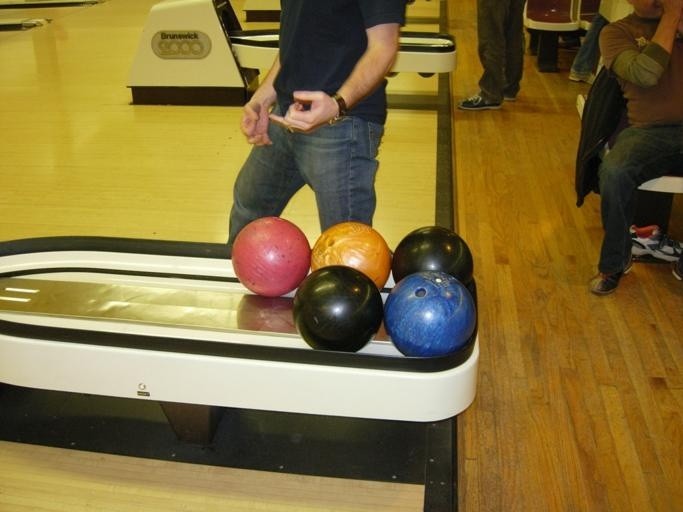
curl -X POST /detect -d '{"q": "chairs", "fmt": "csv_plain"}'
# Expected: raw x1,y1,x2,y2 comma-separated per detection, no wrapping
598,142,683,262
577,56,603,120
523,0,579,72
579,0,602,31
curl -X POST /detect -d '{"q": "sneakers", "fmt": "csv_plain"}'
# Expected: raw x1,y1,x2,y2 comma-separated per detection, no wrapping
568,72,596,85
672,262,682,280
629,224,683,262
503,92,517,100
588,262,632,294
457,90,502,110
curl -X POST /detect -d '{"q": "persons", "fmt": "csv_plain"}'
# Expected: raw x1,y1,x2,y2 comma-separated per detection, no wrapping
225,0,405,247
569,14,609,84
457,0,526,110
587,1,683,296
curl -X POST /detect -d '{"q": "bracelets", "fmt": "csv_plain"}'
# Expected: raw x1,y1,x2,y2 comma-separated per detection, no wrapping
329,91,347,126
